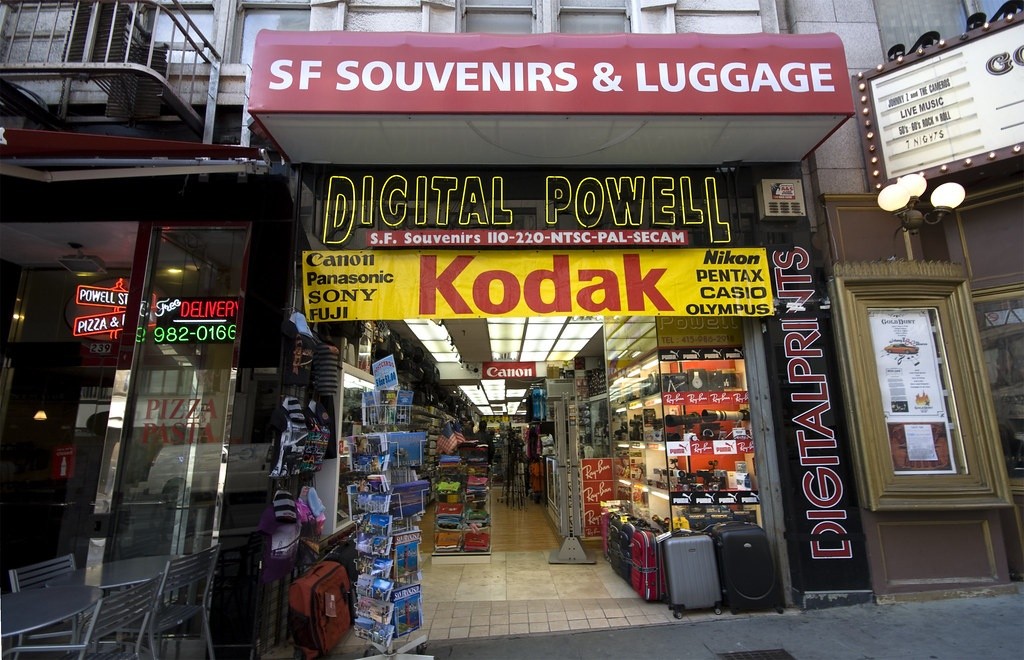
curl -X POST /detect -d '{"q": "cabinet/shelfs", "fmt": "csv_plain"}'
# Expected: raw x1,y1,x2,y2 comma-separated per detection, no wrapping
412,409,444,504
602,316,761,533
431,463,493,557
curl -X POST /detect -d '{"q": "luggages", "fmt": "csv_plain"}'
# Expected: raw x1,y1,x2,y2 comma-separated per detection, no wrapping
712,521,784,614
601,504,669,605
664,529,725,619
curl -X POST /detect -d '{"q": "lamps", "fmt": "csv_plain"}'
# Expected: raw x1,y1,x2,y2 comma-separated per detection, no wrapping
877,174,965,236
56,248,108,276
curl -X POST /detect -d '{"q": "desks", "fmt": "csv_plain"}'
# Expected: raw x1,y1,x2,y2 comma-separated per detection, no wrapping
46,555,188,660
1,585,104,660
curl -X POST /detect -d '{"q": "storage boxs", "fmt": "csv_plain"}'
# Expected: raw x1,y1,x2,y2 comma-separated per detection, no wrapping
547,366,560,379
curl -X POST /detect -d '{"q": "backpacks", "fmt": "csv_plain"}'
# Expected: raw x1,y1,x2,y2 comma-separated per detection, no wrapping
289,560,355,659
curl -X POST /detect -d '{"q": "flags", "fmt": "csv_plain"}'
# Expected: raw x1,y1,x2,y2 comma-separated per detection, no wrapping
436,418,465,456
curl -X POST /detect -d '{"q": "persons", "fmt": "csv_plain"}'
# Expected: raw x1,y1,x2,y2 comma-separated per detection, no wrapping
255,310,339,583
462,415,496,464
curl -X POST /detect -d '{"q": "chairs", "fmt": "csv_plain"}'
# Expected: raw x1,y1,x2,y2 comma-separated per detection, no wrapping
9,553,97,660
117,543,222,660
3,571,166,660
